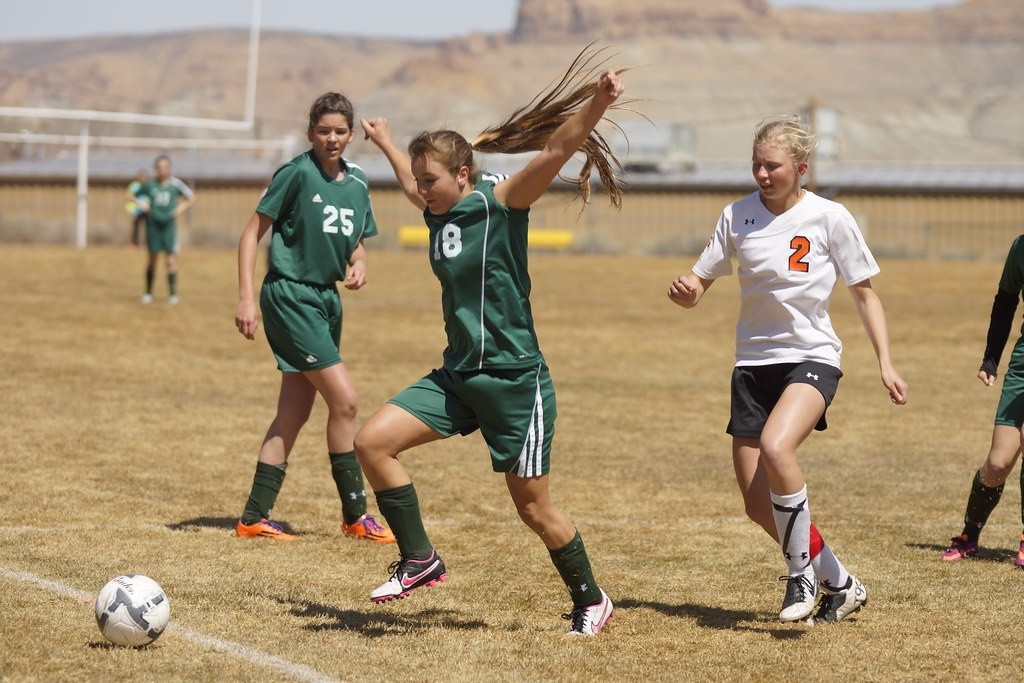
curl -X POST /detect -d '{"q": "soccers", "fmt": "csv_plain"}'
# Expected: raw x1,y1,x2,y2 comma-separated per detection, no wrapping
94,573,171,648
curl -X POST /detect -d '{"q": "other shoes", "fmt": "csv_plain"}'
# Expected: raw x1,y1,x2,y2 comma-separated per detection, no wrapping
168,295,177,305
142,293,152,304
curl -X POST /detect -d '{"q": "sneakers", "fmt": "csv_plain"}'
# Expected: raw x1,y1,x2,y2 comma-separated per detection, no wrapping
370,548,447,604
777,572,818,623
561,587,614,638
813,575,867,622
342,513,397,543
1015,532,1024,567
236,515,293,539
943,534,980,561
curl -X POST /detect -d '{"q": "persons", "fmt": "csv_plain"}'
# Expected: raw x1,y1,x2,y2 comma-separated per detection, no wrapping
124,154,197,305
668,117,907,626
234,92,396,544
352,67,624,641
942,233,1024,567
